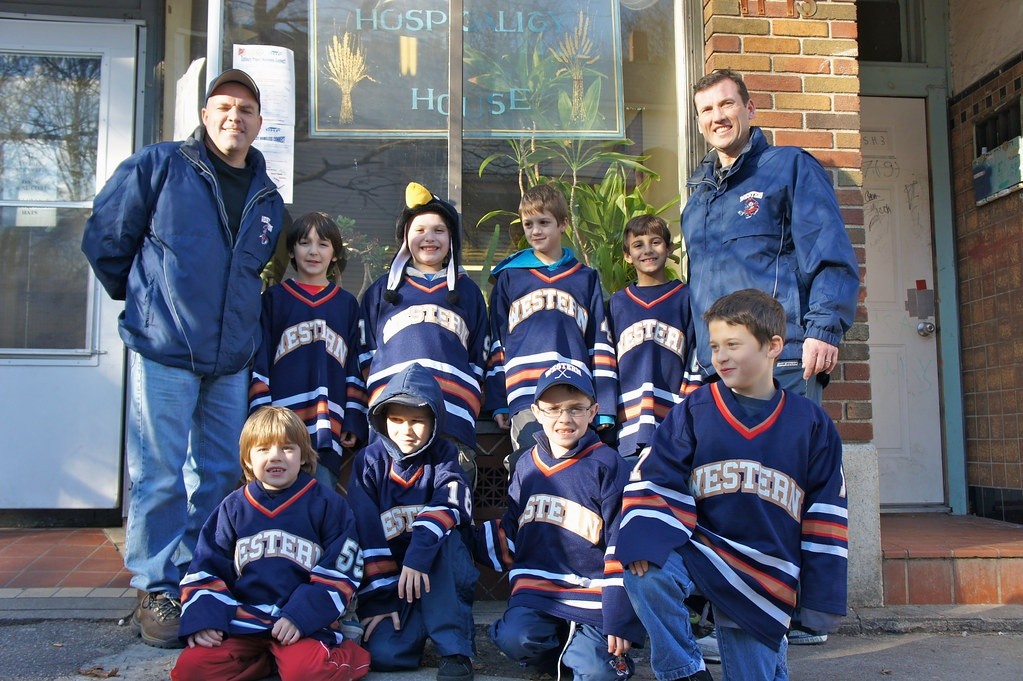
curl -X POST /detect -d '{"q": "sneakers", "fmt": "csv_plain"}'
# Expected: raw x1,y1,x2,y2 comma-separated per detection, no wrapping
130,586,188,649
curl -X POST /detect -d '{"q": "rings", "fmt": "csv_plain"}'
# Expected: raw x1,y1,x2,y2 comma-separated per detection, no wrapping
825,360,832,362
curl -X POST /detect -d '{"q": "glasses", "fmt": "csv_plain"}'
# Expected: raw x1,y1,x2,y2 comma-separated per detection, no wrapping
537,403,595,417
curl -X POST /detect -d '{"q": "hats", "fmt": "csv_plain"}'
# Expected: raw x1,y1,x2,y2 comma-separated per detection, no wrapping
534,362,596,406
384,182,460,305
372,393,428,415
204,68,260,115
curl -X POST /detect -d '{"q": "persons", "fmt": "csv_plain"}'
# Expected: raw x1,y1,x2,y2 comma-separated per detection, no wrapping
170,406,371,681
611,216,703,470
614,289,848,681
248,211,368,492
678,68,860,663
361,182,491,492
347,363,482,681
475,362,647,681
80,69,283,647
487,185,620,482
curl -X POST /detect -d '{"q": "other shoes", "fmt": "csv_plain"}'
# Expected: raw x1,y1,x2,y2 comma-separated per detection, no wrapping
435,654,475,680
689,621,723,662
789,626,828,644
678,660,714,681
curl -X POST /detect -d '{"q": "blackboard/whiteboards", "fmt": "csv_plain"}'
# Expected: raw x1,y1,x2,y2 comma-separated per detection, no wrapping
972,91,1023,206
